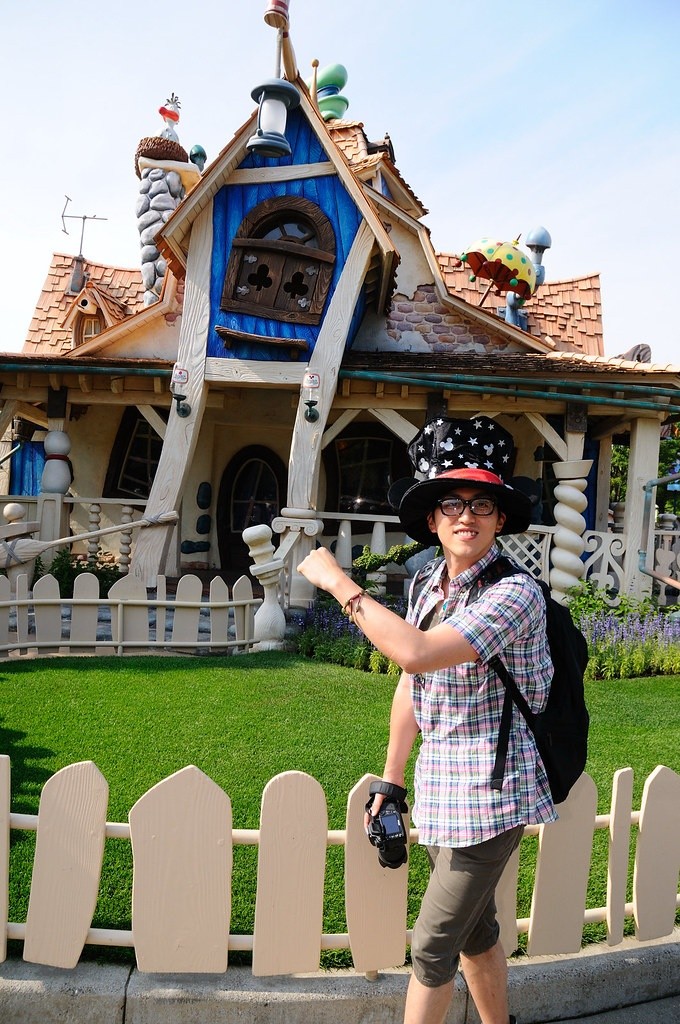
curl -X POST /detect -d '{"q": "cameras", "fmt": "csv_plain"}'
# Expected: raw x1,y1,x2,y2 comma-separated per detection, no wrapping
367,798,408,870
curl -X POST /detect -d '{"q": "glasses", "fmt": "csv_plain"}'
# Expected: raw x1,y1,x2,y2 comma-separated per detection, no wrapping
435,496,498,517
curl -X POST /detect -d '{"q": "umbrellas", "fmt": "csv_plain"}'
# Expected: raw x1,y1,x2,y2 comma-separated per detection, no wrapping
454,234,537,308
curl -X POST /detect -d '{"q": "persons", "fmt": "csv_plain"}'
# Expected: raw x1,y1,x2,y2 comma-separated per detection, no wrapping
296,414,558,1024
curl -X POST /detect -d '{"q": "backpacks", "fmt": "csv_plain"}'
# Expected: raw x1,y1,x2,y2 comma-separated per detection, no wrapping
464,557,590,805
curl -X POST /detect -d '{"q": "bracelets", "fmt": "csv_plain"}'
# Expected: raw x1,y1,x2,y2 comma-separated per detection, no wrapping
341,589,368,623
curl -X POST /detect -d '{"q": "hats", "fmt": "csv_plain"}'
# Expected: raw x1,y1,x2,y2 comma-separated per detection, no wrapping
397,415,535,546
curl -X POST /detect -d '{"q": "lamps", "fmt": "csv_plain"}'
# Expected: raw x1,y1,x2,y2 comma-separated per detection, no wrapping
170,362,194,418
246,25,301,158
304,367,325,422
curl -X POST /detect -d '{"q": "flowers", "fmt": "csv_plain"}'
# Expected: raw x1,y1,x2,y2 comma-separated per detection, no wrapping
570,605,680,679
287,592,408,675
